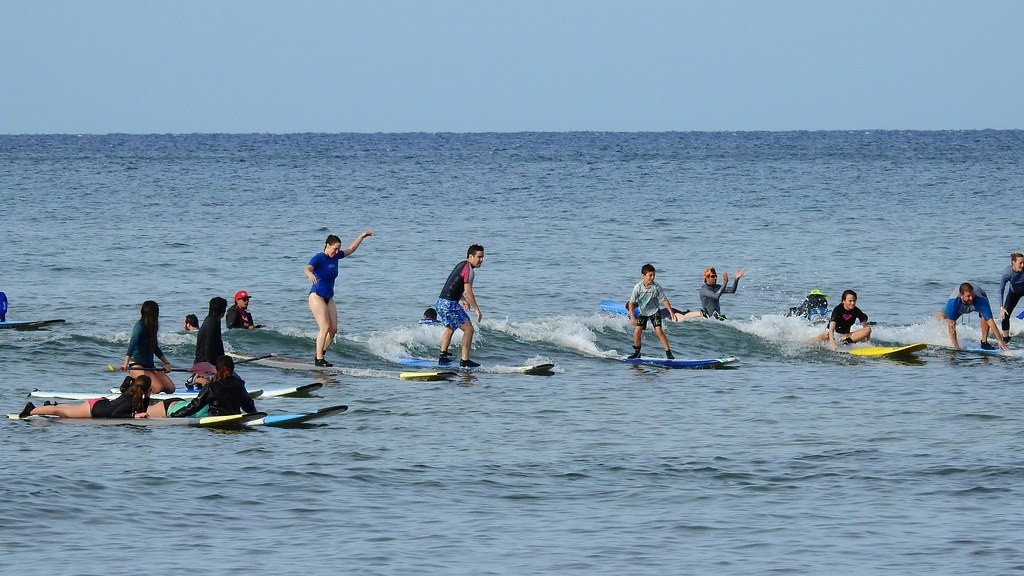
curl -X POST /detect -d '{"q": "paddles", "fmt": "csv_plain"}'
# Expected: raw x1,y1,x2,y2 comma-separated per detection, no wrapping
108,364,217,376
234,352,277,364
255,324,266,328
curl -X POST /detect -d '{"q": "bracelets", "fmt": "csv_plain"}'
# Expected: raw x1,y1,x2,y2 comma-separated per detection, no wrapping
165,363,170,364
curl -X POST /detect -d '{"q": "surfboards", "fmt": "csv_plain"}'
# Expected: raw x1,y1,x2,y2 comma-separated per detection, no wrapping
4,412,268,425
611,355,737,368
107,381,324,398
226,352,458,377
598,300,639,313
28,387,266,399
236,403,350,425
400,357,553,373
950,347,1024,359
851,342,928,356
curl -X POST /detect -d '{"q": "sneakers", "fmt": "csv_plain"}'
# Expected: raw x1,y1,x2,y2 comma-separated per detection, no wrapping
460,359,481,367
120,376,134,393
627,345,642,358
315,357,334,367
1003,336,1011,342
18,403,36,418
666,349,674,359
44,400,51,406
980,340,995,350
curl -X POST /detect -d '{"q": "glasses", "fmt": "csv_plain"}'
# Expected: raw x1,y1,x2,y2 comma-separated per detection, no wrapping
708,275,717,278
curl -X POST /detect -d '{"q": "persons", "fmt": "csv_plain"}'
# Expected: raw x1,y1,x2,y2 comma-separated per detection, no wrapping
185,297,241,391
184,314,200,331
934,281,1011,352
800,289,872,352
699,266,746,318
418,307,441,324
119,301,176,394
226,290,254,330
624,301,727,322
998,251,1024,344
435,245,485,368
135,356,257,419
18,375,152,419
628,263,678,360
304,229,372,367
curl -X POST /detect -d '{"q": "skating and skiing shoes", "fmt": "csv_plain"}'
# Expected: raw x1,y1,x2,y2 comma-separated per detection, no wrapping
437,357,453,365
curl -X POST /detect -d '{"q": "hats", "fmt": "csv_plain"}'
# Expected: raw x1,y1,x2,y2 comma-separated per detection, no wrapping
235,290,252,302
